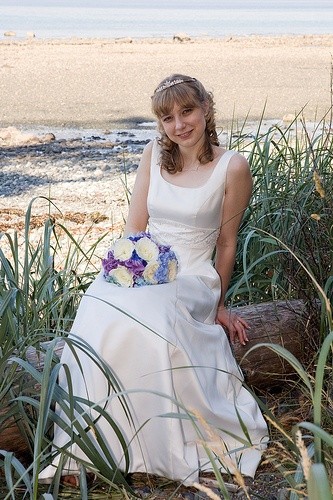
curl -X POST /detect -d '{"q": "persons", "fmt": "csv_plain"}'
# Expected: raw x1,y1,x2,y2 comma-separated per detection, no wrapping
49,74,252,457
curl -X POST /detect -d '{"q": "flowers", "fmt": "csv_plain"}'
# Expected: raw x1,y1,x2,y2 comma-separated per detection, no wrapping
102,234,178,289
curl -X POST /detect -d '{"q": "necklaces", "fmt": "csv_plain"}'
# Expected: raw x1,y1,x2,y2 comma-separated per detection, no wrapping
178,158,200,171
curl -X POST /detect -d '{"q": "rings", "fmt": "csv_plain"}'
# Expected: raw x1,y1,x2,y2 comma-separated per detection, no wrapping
236,320,239,324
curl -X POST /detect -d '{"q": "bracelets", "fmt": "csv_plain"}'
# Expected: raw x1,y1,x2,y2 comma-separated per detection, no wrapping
217,305,229,313
